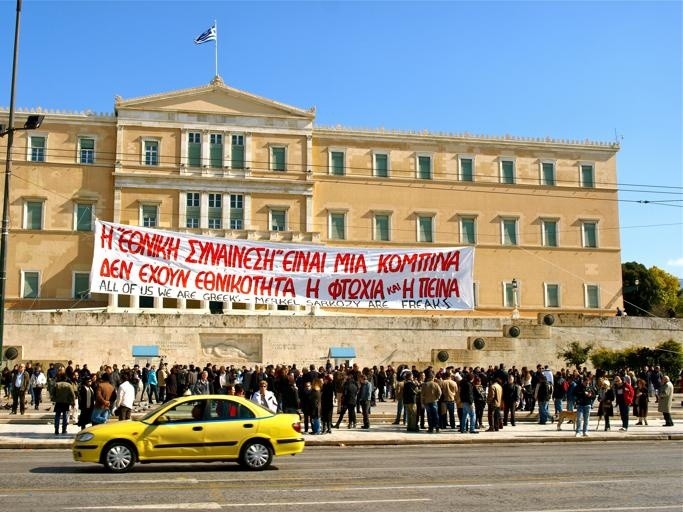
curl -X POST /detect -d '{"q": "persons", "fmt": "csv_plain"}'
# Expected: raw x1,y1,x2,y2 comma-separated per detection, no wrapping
668,306,676,318
614,306,623,316
3,359,388,435
671,366,682,386
385,363,674,438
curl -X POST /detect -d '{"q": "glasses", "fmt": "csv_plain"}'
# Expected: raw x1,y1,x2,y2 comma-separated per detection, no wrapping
260,385,266,388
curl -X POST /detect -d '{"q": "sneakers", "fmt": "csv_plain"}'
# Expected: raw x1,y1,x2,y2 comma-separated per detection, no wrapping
575,419,674,437
552,416,555,423
20,410,24,415
9,412,16,414
309,421,372,435
538,421,546,424
393,417,517,432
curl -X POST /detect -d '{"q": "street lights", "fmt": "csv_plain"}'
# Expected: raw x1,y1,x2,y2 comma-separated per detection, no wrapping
510,277,520,319
0,115,46,372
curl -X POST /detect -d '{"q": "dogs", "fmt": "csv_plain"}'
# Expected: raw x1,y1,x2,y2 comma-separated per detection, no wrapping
552,410,578,431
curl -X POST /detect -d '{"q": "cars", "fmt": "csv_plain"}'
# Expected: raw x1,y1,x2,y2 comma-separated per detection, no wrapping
71,393,305,473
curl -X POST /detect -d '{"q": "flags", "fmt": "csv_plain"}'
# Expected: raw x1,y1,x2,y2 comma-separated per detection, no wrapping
191,24,216,46
